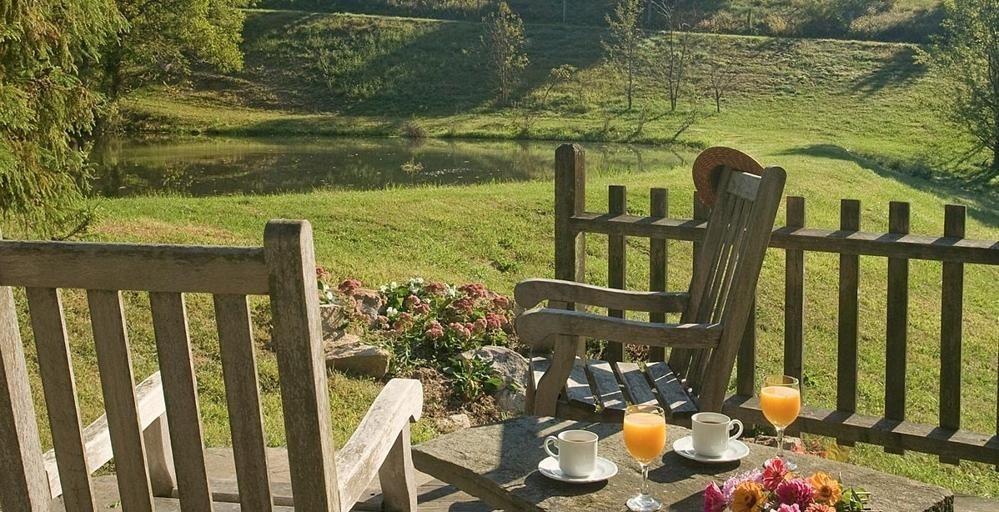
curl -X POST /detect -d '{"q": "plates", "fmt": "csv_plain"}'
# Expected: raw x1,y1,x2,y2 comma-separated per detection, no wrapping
538,456,619,484
672,433,750,463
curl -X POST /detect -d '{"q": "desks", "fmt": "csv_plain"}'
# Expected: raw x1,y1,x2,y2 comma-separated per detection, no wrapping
410,414,956,511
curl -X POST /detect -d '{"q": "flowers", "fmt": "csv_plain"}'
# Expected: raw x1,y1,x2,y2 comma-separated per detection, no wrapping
702,456,873,511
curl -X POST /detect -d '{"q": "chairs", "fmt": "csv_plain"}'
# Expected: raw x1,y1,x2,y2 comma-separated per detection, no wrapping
3,218,424,512
516,163,786,429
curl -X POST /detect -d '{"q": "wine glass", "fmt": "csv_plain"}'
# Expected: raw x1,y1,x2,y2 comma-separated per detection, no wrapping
622,404,667,511
760,374,801,472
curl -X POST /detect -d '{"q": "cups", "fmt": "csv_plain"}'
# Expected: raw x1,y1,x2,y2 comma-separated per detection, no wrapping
691,410,744,458
543,429,599,477
